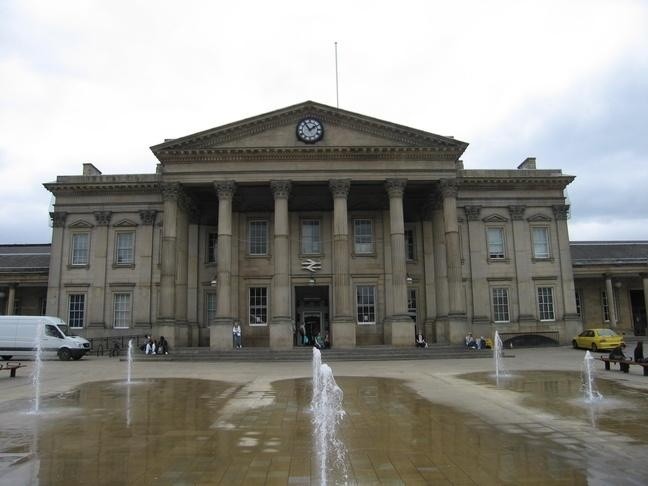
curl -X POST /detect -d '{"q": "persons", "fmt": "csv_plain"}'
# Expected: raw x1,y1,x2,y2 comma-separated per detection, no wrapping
634,341,648,363
232,319,242,350
609,341,628,359
140,334,169,355
465,332,474,347
416,330,425,347
299,326,331,349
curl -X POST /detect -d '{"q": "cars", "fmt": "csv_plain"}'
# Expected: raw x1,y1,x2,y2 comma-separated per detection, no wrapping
571,328,626,352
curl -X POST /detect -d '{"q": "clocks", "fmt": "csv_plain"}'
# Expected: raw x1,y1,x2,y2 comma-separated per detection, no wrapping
295,118,324,144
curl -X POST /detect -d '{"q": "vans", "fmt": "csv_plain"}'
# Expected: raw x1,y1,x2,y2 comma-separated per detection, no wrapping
0,315,92,361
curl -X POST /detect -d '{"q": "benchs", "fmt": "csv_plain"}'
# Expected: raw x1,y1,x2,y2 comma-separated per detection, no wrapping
594,356,648,376
